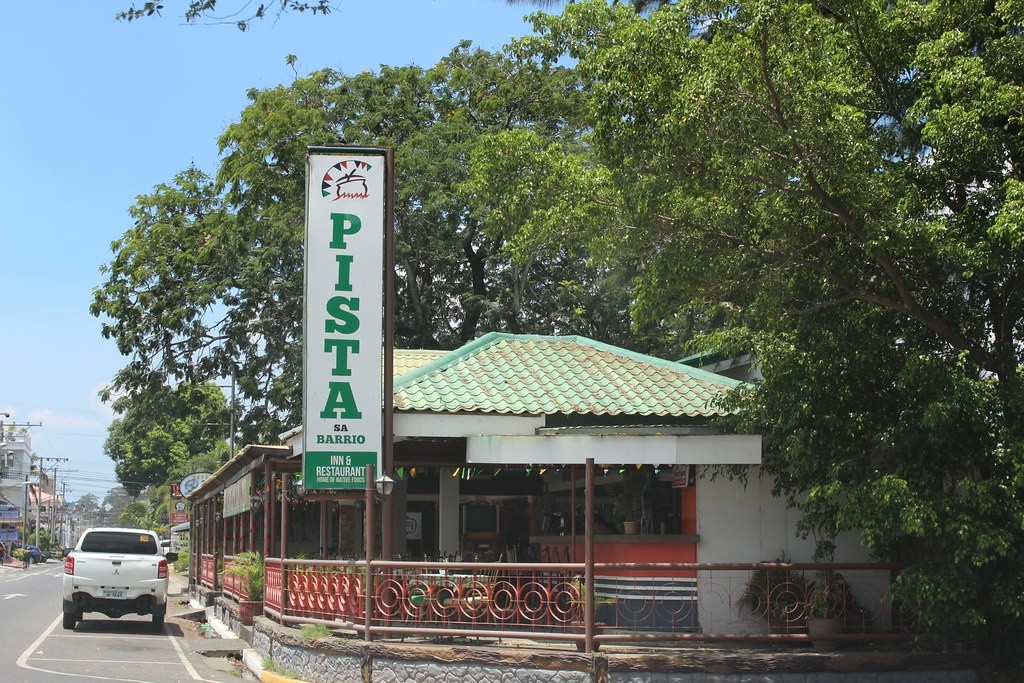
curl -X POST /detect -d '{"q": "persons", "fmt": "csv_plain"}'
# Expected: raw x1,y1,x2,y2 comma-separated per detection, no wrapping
593,514,617,534
23,547,31,569
0,545,5,560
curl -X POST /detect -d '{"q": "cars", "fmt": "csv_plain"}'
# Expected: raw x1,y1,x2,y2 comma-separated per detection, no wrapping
14,543,42,563
40,554,47,561
159,539,173,556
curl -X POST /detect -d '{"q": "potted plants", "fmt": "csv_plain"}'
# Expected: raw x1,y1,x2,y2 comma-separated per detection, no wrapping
564,576,618,652
734,551,811,635
795,494,853,651
223,548,266,626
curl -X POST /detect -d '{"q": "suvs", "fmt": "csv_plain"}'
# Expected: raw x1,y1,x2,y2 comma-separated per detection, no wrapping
60,527,178,633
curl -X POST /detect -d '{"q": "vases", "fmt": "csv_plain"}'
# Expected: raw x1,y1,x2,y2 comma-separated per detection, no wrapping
622,520,643,536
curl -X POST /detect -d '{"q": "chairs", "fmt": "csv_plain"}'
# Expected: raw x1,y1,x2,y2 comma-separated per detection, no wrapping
324,542,575,646
